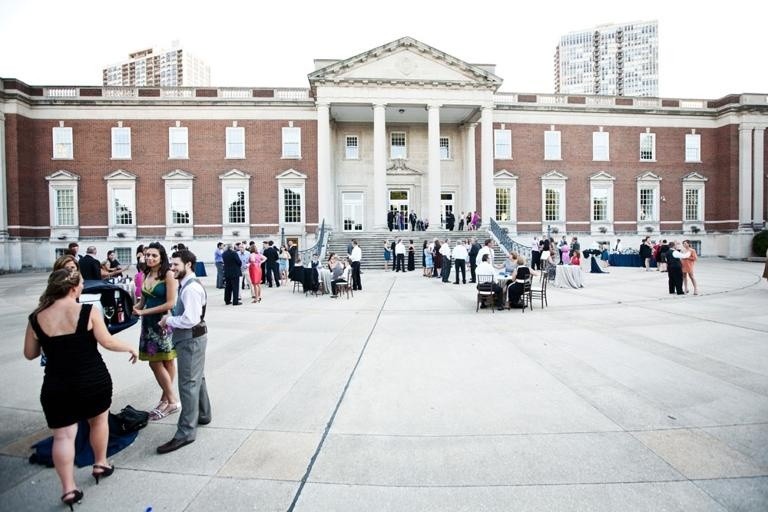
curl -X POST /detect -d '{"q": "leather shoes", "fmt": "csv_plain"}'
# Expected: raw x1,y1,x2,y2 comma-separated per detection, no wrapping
157,438,194,453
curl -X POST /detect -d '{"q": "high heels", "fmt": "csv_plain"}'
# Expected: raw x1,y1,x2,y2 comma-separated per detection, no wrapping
61,490,83,511
93,466,114,484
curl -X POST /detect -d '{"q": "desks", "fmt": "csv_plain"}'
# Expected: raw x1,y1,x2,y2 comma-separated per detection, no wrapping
114,281,138,305
608,252,657,267
556,265,583,289
196,261,206,277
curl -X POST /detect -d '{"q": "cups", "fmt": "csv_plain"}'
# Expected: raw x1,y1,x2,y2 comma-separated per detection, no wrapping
118,276,121,282
121,279,125,283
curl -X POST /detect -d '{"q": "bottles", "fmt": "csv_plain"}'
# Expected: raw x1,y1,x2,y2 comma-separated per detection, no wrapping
113,290,122,321
126,275,129,284
116,303,125,323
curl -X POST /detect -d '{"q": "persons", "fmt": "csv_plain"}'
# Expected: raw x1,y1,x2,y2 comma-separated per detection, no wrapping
346,239,362,290
601,242,609,267
40,255,79,366
665,241,691,295
384,239,416,272
131,243,179,420
423,237,482,284
69,242,146,298
474,238,541,308
308,252,351,298
446,210,481,231
215,240,296,305
171,243,185,253
156,250,212,453
531,235,581,270
680,239,698,295
639,237,682,272
23,268,137,512
387,208,429,231
612,239,621,251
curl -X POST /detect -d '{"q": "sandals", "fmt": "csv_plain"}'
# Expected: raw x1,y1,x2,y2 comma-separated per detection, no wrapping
150,400,179,420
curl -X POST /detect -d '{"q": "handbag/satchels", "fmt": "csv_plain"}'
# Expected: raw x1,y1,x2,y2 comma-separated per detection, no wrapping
110,405,150,435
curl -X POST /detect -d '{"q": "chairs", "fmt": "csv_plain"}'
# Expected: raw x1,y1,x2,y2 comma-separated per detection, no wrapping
293,266,353,299
476,273,549,313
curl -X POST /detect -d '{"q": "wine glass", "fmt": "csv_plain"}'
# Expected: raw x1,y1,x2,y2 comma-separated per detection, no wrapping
103,306,115,327
